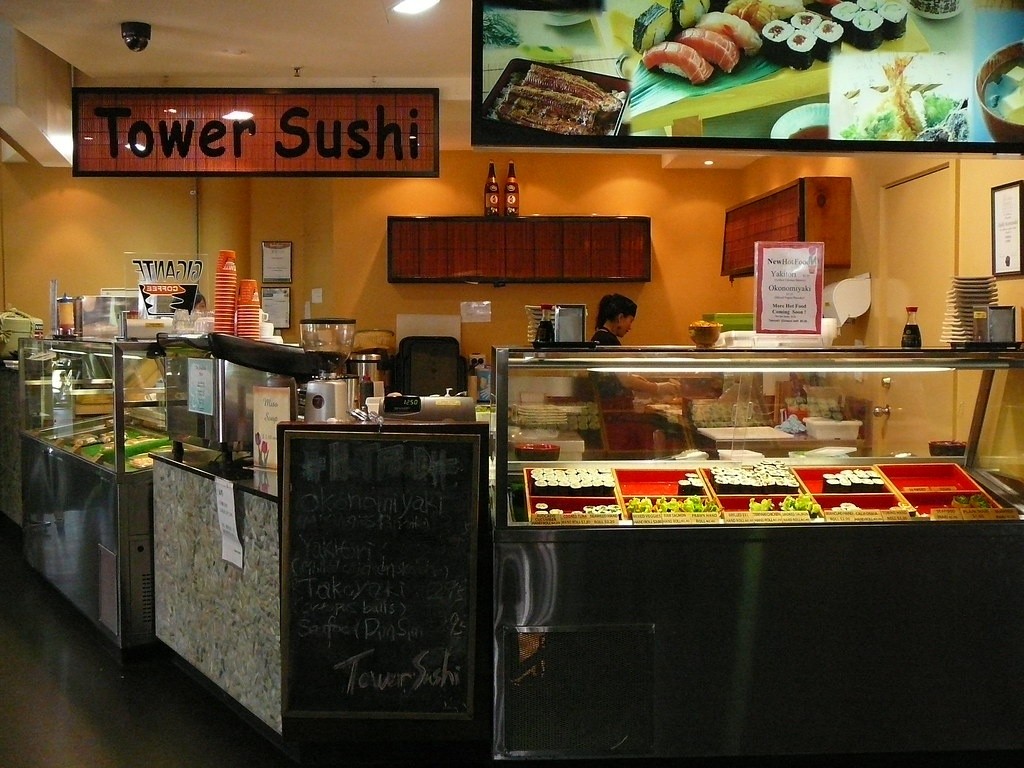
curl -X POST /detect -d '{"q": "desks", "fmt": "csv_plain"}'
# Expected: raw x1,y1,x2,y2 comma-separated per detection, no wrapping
644,397,877,457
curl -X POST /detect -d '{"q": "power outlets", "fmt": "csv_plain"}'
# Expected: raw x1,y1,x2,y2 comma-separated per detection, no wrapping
469,354,486,368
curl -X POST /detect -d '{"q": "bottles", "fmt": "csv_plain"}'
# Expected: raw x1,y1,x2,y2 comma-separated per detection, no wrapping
360,373,374,411
534,303,554,343
901,306,922,348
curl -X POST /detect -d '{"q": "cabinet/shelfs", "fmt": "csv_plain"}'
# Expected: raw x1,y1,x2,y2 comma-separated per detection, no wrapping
720,175,852,283
386,215,652,282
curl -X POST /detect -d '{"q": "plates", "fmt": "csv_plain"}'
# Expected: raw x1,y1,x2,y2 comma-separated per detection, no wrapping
939,274,999,343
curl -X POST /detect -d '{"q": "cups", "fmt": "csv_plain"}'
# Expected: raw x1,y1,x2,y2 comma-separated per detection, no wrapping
213,249,260,339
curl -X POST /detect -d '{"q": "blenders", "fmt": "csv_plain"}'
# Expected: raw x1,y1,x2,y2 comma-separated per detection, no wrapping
299,316,357,425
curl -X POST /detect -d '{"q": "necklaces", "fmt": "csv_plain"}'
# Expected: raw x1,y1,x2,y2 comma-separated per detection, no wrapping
600,326,610,332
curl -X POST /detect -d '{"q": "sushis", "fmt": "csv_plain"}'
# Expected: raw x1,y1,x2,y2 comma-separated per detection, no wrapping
530,462,993,520
612,1,909,81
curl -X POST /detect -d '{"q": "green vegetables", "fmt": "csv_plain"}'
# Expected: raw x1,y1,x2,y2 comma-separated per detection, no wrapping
927,93,958,126
841,114,903,139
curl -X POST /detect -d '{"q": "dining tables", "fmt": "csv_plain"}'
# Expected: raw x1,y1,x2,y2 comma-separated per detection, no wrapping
482,7,933,137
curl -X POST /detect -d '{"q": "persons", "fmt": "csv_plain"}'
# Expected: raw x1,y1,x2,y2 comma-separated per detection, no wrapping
586,294,680,451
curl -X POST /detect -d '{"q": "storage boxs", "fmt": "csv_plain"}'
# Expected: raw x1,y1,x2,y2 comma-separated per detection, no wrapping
523,464,1004,520
803,415,864,441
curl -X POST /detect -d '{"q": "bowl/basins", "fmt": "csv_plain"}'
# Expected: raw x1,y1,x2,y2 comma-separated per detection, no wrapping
688,326,721,349
514,444,560,461
